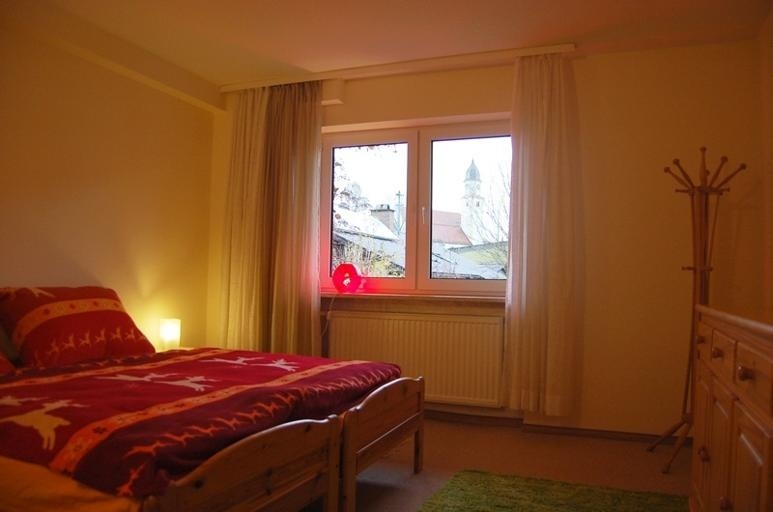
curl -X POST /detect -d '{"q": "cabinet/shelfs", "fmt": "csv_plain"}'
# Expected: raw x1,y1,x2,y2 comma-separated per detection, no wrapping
689,303,772,512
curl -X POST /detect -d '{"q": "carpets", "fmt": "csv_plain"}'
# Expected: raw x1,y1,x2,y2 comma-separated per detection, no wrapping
418,471,689,511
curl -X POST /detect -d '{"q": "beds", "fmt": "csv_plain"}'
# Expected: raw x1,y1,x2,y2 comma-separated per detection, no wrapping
0,286,425,511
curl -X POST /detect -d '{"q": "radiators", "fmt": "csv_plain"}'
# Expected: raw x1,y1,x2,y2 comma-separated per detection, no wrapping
323,308,506,412
